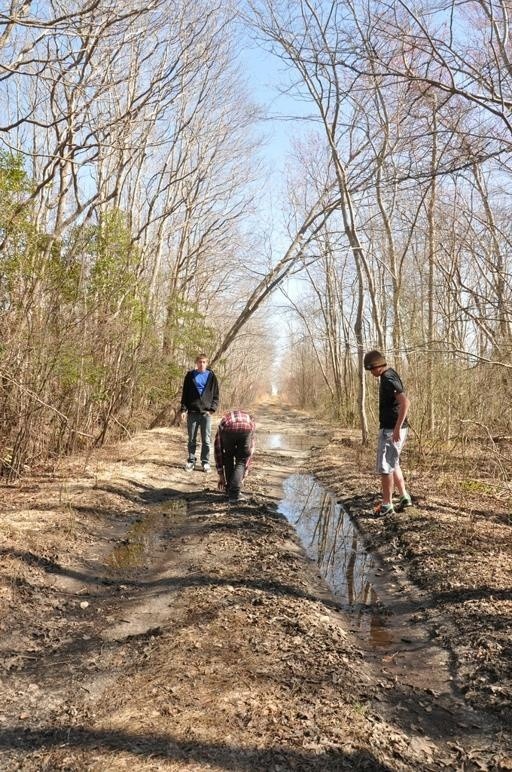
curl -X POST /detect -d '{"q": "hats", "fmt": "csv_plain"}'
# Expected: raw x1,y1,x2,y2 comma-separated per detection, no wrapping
364,351,387,371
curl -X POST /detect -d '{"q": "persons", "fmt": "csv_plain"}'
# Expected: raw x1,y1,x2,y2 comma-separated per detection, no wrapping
363,351,409,518
179,352,221,473
213,410,257,504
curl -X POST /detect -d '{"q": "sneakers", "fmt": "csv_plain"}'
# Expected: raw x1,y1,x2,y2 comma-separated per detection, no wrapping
203,463,211,473
228,496,239,504
185,462,194,472
398,494,412,511
373,503,395,518
